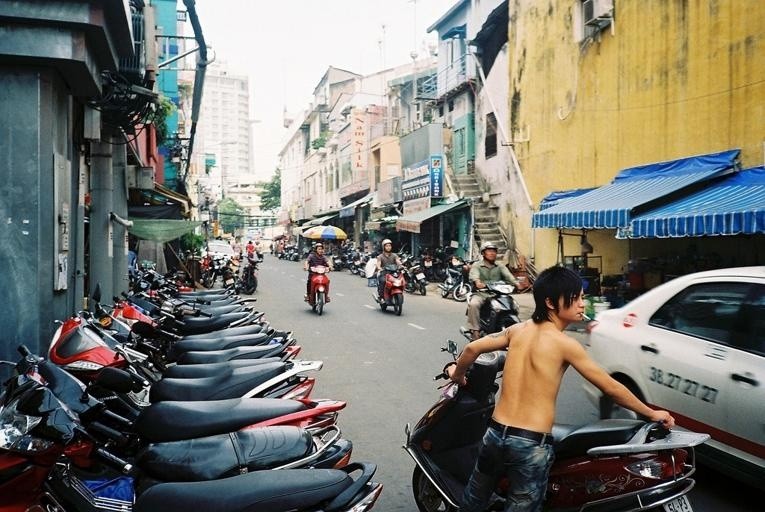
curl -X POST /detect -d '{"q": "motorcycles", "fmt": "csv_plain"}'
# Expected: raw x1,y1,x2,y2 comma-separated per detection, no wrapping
458,282,522,353
333,248,372,277
203,256,262,294
303,265,330,316
401,256,429,296
371,263,406,315
436,266,472,302
401,338,712,512
46,274,301,343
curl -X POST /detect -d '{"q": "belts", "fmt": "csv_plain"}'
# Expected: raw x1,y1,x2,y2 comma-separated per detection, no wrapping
486,417,555,447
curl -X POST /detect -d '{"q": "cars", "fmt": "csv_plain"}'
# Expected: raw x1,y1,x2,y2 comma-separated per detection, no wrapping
201,241,238,268
581,264,765,474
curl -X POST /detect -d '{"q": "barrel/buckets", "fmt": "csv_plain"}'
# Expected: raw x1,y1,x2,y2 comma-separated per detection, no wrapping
593,303,611,319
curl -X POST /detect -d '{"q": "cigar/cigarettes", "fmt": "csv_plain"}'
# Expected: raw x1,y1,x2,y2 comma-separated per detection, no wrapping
579,311,592,324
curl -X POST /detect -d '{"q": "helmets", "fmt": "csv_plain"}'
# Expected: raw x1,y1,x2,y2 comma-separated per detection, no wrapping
315,242,323,249
480,241,500,252
381,238,393,245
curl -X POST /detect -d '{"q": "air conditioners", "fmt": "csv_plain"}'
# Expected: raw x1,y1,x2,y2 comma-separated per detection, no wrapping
583,1,614,25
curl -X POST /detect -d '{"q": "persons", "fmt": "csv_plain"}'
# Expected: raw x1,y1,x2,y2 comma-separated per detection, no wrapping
303,243,334,302
223,234,264,261
444,263,676,512
364,252,380,280
375,239,407,304
465,241,523,341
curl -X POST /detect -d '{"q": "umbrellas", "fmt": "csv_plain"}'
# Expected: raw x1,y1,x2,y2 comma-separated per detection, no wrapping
301,225,347,238
